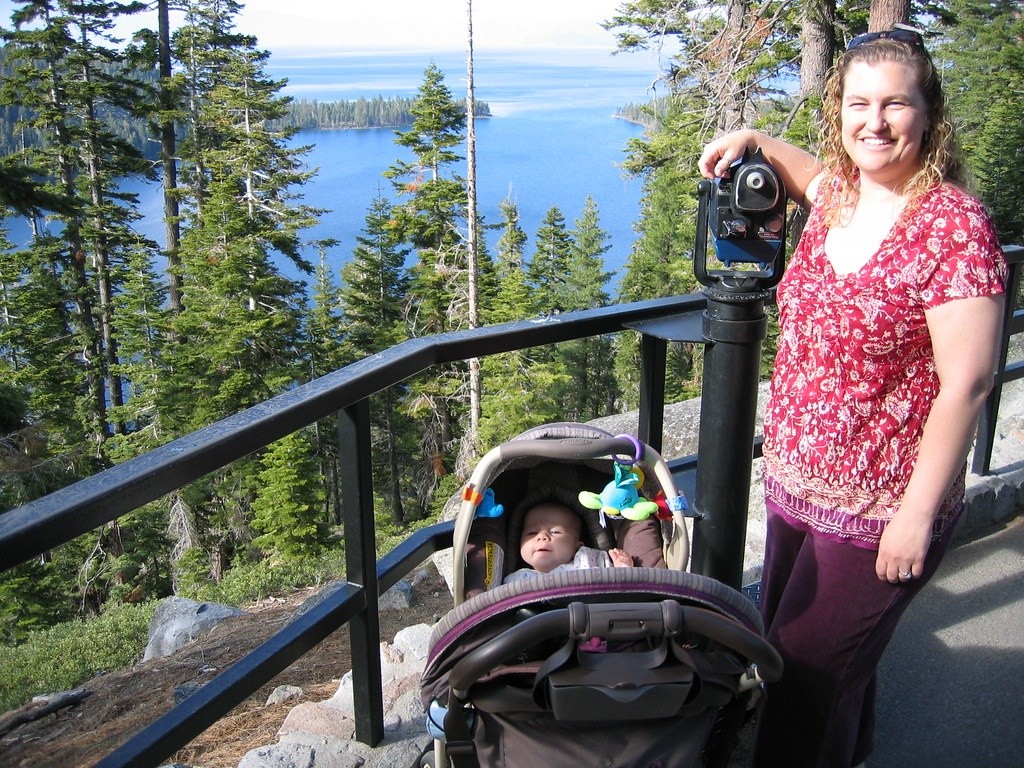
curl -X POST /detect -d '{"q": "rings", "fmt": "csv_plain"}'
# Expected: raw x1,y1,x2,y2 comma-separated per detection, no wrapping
902,570,912,579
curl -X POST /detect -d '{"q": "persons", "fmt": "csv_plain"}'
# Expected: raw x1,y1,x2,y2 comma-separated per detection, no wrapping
697,36,1008,768
466,501,634,599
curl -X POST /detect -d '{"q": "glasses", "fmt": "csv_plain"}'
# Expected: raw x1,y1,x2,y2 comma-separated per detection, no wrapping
844,29,926,57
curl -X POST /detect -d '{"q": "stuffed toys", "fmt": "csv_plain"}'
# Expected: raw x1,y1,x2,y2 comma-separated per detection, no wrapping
579,466,659,521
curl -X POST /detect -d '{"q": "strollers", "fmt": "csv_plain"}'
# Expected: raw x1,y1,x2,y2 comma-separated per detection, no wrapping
408,421,786,768
